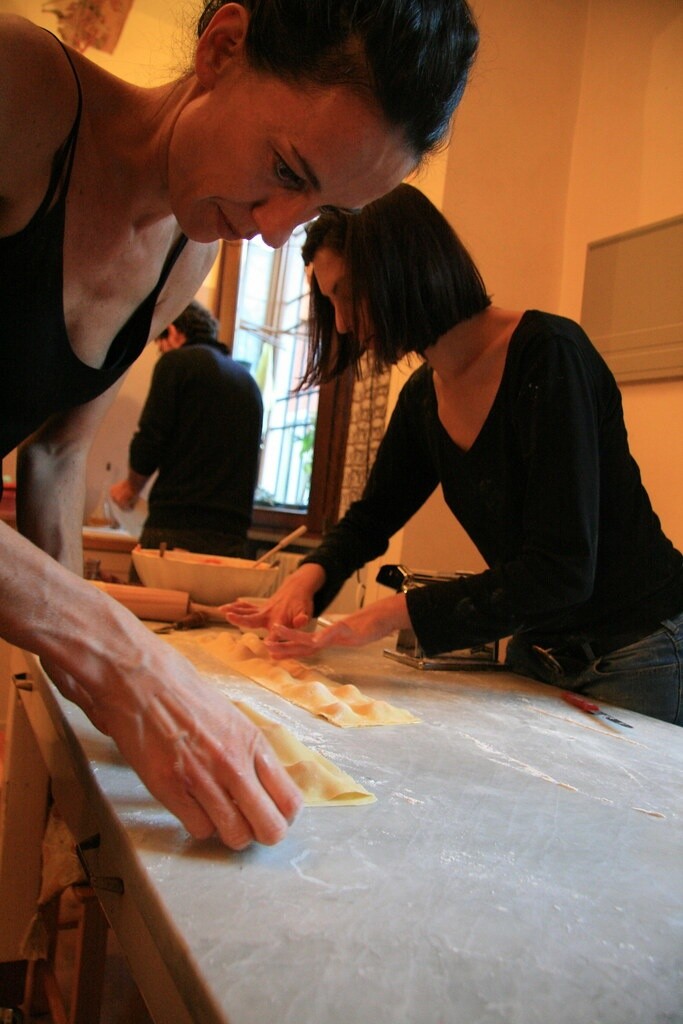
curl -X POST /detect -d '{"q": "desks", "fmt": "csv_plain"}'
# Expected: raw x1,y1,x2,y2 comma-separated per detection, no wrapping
14,613,682,1024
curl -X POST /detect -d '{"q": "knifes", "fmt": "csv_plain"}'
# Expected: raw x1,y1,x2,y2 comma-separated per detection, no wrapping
560,688,636,730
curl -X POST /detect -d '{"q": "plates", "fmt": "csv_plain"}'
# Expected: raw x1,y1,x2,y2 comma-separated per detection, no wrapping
109,495,148,538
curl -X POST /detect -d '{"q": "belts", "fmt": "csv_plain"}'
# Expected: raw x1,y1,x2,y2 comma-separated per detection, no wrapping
526,597,682,684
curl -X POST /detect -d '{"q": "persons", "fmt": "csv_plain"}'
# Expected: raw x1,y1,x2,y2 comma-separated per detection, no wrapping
0,1,481,856
225,184,682,723
110,301,263,584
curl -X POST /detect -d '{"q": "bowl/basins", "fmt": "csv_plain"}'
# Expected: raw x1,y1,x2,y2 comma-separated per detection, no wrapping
131,548,279,606
